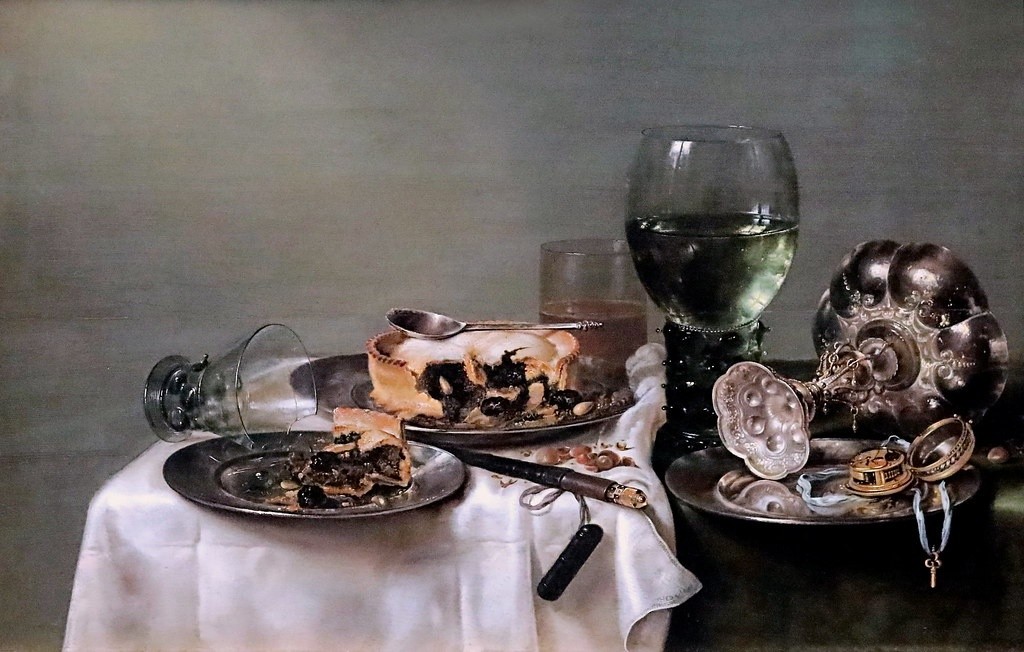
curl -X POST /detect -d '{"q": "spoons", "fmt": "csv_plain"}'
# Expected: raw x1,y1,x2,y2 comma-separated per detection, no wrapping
386,308,604,340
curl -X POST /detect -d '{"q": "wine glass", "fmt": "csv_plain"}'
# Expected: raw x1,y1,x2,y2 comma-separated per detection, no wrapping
711,239,1008,479
625,125,800,453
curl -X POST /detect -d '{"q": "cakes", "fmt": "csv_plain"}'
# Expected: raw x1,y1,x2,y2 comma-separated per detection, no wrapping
365,319,581,426
272,405,412,506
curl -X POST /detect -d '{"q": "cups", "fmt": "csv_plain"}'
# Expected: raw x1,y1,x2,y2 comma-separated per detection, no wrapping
540,238,647,362
143,324,318,448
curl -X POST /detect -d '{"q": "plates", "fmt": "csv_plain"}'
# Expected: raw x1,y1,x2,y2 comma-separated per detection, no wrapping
291,354,636,445
665,439,982,525
163,430,465,519
